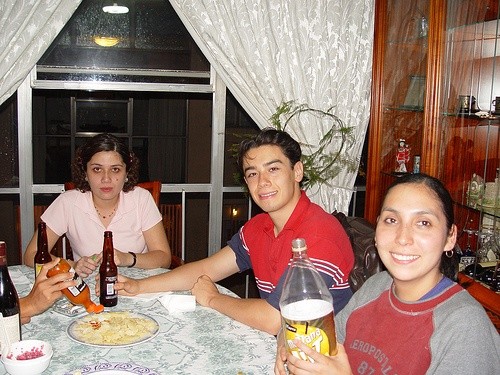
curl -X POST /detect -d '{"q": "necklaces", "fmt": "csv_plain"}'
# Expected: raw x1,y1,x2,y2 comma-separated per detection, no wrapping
92,200,116,219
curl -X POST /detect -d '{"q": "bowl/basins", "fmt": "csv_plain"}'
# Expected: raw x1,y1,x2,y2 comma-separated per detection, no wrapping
1,340,53,375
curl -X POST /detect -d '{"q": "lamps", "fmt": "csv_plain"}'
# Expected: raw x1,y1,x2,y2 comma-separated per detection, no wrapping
101,3,128,14
91,35,120,47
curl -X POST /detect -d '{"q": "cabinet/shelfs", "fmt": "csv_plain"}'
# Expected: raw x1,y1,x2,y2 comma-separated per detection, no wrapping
364,0,500,331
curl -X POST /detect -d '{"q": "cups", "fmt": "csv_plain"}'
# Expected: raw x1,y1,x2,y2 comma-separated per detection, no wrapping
491,96,500,115
459,94,475,114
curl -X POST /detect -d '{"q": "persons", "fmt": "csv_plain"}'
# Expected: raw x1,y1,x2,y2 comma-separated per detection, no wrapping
394,140,411,175
95,127,355,336
275,173,500,375
17,258,78,325
25,133,172,278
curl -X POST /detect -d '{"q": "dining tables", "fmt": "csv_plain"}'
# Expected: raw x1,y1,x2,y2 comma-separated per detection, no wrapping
0,265,289,375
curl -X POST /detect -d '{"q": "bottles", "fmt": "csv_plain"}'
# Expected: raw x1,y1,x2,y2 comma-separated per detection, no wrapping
99,231,119,307
278,237,337,363
33,222,51,282
46,259,103,314
0,241,22,356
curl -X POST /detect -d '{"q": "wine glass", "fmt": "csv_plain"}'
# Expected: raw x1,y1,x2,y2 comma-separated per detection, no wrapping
460,216,500,284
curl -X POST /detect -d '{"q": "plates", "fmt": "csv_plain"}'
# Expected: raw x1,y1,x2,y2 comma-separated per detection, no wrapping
66,311,159,348
64,361,158,375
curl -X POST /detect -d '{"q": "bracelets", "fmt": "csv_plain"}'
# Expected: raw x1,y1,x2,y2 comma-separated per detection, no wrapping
128,251,136,268
